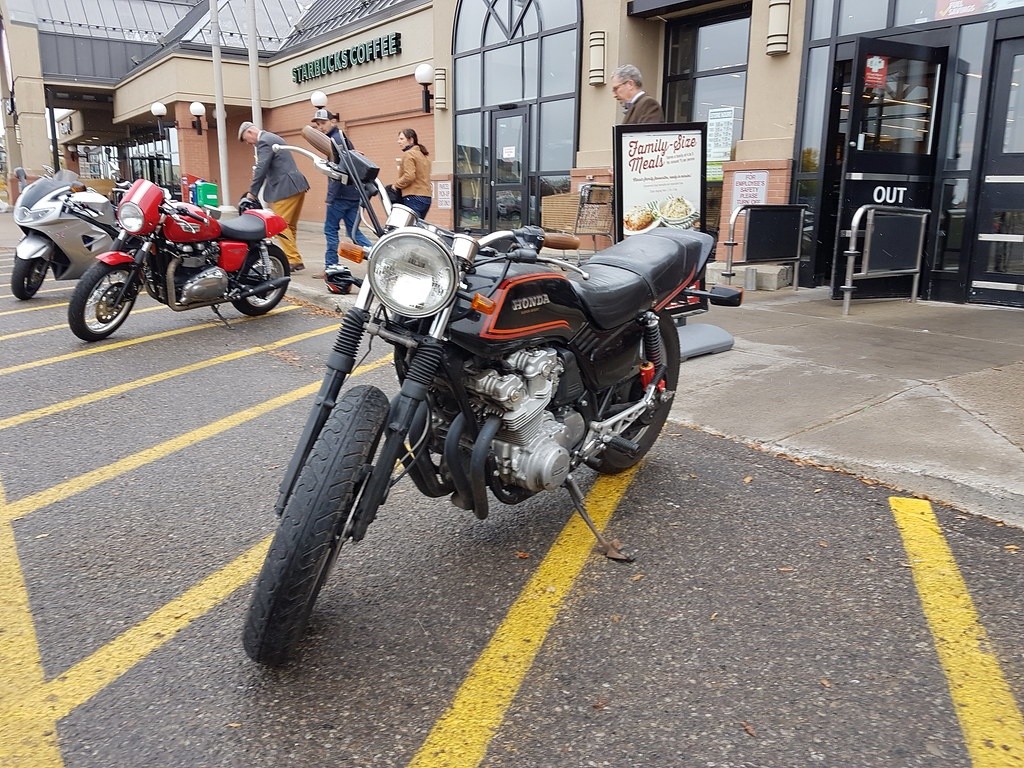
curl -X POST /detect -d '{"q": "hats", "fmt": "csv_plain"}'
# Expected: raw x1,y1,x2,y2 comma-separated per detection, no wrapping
238,121,255,143
311,109,333,122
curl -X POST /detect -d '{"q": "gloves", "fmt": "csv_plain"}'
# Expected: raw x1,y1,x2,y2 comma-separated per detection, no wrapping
324,194,334,206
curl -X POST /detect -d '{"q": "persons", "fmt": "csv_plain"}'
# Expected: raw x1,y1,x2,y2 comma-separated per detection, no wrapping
611,64,665,125
237,121,310,273
391,128,432,220
310,109,374,279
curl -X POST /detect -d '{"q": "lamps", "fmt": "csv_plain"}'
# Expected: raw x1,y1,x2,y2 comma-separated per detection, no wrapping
435,67,448,111
414,63,434,113
79,147,90,162
151,102,178,136
589,30,607,87
67,145,76,161
765,0,792,57
311,91,340,122
105,148,120,161
209,109,227,128
190,102,206,135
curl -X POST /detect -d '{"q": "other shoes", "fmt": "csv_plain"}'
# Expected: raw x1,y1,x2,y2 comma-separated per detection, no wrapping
312,273,324,279
289,263,304,271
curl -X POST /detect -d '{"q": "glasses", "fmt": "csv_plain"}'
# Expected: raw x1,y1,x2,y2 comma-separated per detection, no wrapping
612,80,628,93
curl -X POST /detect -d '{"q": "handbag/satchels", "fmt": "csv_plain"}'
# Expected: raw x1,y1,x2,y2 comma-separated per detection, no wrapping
385,185,403,204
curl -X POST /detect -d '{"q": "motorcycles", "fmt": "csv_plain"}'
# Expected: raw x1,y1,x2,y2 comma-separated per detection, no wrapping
241,122,745,668
9,165,292,343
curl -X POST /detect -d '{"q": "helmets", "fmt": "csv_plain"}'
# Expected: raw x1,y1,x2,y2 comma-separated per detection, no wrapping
238,191,263,216
324,264,353,294
